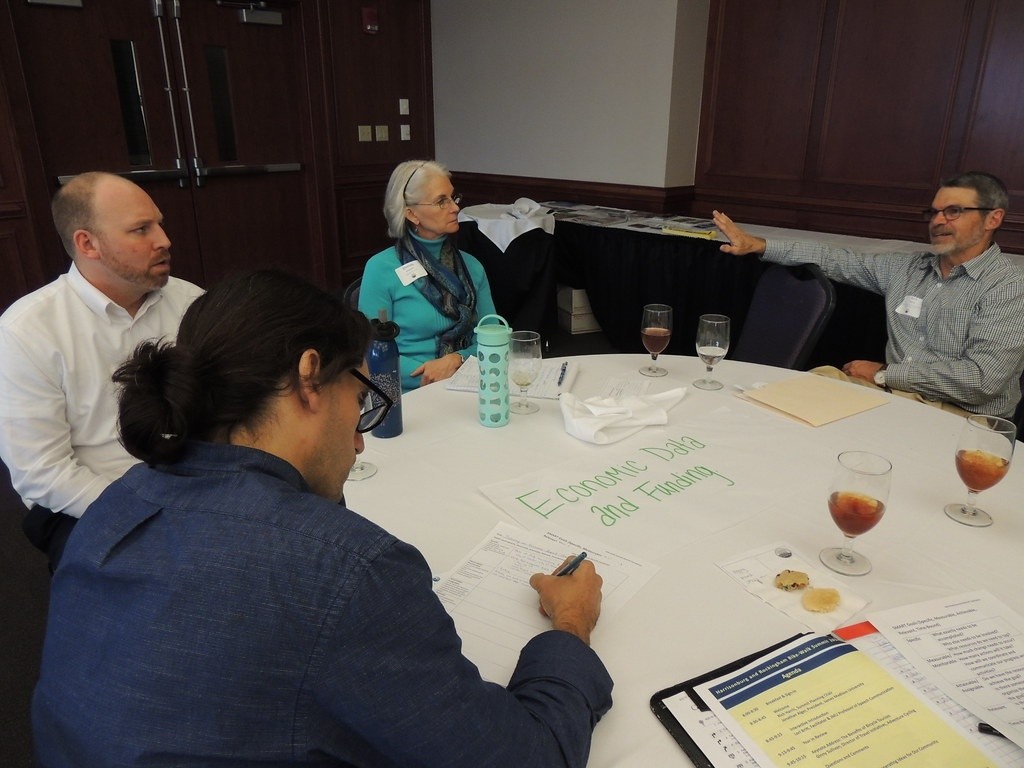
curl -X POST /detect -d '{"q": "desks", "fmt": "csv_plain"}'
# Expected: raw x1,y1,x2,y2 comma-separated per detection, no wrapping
342,352,1024,768
542,200,887,368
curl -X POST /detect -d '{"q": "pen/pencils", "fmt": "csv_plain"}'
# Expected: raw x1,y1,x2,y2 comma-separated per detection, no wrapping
978,723,1004,736
557,361,567,386
556,552,587,576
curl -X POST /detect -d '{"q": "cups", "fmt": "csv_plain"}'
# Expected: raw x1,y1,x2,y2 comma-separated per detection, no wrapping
473,313,513,428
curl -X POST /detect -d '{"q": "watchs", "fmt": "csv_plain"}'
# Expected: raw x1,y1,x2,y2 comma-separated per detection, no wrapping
873,364,889,389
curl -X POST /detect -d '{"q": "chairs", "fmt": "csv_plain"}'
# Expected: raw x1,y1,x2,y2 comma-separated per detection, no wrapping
731,261,836,369
345,219,557,357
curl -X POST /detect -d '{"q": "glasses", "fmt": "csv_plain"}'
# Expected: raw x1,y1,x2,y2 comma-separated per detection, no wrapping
921,205,998,221
345,366,394,433
405,192,462,209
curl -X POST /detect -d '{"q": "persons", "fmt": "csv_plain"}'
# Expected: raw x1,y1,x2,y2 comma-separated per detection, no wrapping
358,160,501,394
713,172,1024,432
0,171,208,582
30,267,614,768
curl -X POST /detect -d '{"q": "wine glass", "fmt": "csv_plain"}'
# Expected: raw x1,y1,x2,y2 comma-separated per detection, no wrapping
509,330,543,415
342,453,377,481
943,415,1017,528
639,304,672,376
818,449,893,575
692,314,731,391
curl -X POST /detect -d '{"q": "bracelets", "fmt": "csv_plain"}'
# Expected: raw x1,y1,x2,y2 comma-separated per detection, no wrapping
456,352,464,366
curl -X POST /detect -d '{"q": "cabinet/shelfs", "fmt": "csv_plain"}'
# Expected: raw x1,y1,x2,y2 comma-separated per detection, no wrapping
694,0,1024,255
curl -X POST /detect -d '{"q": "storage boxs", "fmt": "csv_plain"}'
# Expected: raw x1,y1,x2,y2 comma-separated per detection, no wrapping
557,285,603,336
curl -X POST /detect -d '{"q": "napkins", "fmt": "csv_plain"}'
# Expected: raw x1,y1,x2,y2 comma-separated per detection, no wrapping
559,387,689,444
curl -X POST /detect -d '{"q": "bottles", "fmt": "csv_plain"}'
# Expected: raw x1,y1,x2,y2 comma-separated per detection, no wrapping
365,308,403,438
